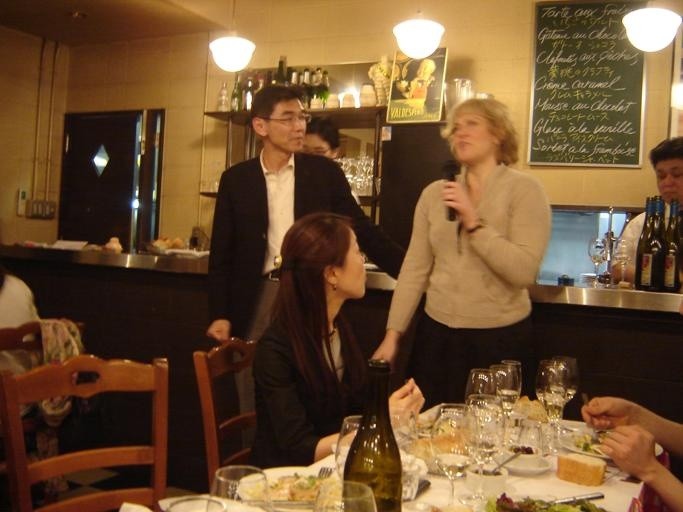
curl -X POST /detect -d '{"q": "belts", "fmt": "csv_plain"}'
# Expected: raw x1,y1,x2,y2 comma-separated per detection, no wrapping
262,267,281,282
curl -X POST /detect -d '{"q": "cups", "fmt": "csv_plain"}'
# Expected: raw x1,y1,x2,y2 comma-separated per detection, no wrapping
515,420,541,450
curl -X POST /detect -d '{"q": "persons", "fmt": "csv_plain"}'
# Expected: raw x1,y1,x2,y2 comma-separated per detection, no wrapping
205,85,408,452
0,274,44,417
581,395,682,512
300,117,342,163
372,98,552,412
251,213,425,469
610,137,682,285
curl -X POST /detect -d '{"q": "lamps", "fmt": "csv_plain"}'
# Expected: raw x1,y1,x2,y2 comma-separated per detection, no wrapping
622,1,681,54
209,0,255,74
393,0,446,59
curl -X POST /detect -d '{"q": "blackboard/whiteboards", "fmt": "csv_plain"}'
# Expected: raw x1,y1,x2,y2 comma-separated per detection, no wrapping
527,0,646,167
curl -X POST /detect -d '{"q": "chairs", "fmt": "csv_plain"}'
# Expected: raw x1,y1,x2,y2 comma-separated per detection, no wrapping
0,357,167,512
0,324,80,498
194,339,256,493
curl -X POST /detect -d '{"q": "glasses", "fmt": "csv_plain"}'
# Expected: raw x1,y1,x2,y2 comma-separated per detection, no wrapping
256,113,311,127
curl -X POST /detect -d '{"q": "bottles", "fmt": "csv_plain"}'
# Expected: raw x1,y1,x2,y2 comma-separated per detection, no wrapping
342,359,402,512
230,59,330,111
634,196,653,289
641,196,665,292
217,82,230,111
664,197,682,293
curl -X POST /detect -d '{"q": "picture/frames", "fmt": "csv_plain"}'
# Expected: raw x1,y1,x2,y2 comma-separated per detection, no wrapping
387,48,448,122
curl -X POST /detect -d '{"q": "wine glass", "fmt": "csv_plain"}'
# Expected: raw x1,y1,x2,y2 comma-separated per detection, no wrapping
313,481,378,511
488,366,515,426
500,360,521,411
432,411,482,511
165,497,228,512
549,357,575,417
468,423,501,506
465,370,495,415
587,239,606,290
334,417,364,481
534,361,566,439
614,239,632,289
460,394,505,506
206,466,271,512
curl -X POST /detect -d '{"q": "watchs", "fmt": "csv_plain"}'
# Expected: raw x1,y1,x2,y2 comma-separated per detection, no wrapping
466,217,485,234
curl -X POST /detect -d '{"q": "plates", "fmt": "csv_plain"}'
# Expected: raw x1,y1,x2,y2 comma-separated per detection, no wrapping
557,427,662,463
238,466,319,511
495,455,551,475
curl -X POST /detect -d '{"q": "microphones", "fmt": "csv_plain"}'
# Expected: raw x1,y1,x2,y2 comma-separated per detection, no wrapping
441,158,459,222
